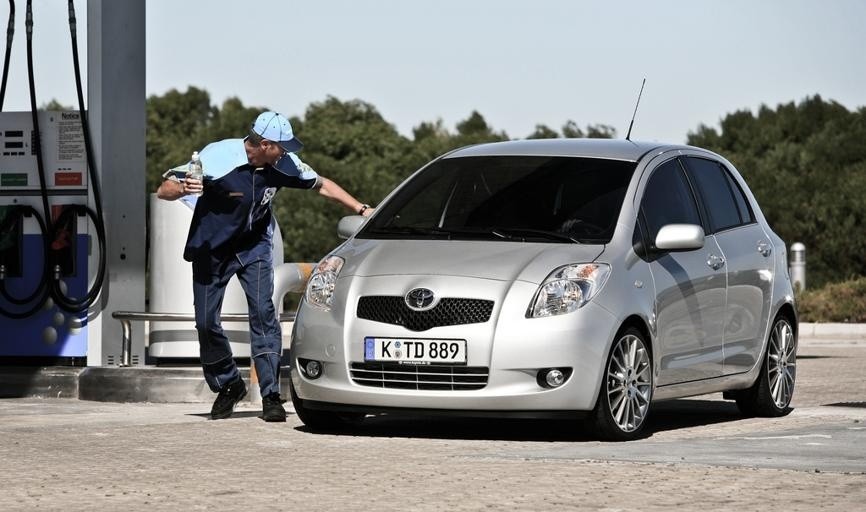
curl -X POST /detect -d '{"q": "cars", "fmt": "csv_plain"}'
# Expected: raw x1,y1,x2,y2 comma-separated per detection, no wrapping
289,137,797,433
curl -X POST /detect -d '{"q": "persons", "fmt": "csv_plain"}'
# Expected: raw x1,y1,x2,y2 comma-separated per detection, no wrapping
156,110,378,421
557,215,585,234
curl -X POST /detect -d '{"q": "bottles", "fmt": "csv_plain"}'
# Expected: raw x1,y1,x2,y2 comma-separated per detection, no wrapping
189,152,203,195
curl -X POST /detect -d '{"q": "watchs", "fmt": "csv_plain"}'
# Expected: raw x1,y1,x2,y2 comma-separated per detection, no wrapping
358,204,370,215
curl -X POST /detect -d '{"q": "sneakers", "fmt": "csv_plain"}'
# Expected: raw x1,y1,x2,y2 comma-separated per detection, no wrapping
211,374,247,419
262,396,287,423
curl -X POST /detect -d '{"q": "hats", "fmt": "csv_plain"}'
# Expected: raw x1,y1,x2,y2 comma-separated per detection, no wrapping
253,111,305,154
